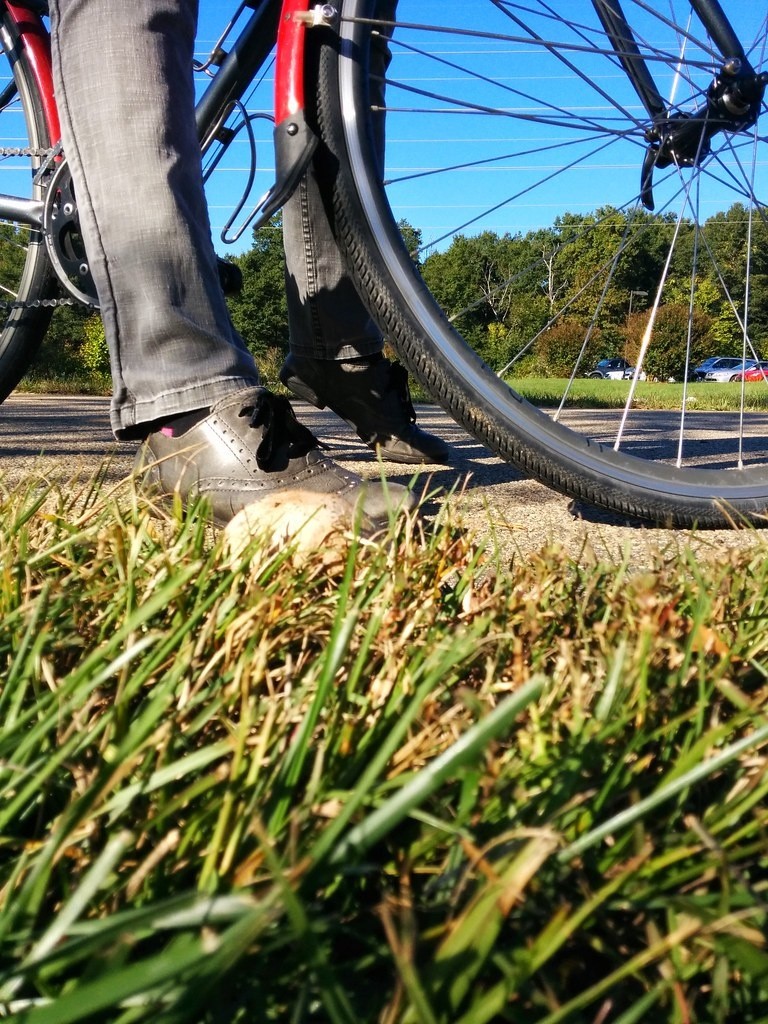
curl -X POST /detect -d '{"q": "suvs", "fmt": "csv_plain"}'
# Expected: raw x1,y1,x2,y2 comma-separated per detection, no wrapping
585,358,629,380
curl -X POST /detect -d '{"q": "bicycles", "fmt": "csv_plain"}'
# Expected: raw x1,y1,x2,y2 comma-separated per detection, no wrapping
1,1,768,522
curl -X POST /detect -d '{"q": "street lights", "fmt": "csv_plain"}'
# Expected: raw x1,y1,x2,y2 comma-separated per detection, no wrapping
624,291,648,379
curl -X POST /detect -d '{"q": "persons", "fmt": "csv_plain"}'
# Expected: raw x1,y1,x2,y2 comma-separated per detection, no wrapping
49,1,453,549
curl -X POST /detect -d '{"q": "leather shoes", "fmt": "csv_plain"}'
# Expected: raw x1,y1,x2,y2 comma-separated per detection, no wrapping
132,386,420,538
286,352,453,466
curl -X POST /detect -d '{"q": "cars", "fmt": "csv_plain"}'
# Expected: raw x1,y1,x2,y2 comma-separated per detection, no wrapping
694,356,742,380
704,361,760,382
735,362,768,382
605,365,677,383
